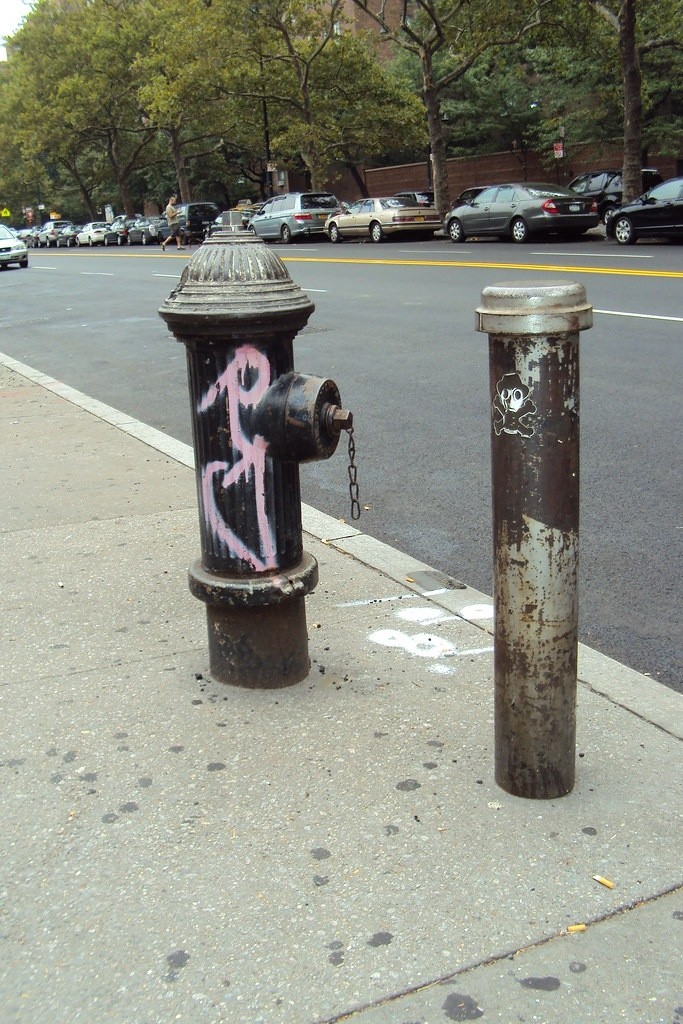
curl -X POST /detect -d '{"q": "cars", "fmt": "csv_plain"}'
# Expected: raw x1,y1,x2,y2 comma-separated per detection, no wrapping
0,192,342,269
324,190,444,244
607,176,682,246
443,181,599,243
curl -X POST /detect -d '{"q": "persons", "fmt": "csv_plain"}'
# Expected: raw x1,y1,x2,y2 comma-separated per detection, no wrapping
339,201,350,209
160,197,184,251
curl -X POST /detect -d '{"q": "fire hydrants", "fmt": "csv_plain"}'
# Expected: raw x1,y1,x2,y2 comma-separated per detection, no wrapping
157,211,361,689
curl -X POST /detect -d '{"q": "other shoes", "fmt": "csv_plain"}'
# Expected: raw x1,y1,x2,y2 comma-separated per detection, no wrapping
177,246,186,250
160,242,165,252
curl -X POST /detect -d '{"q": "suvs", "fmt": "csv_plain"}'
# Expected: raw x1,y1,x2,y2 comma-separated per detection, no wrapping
567,168,664,225
